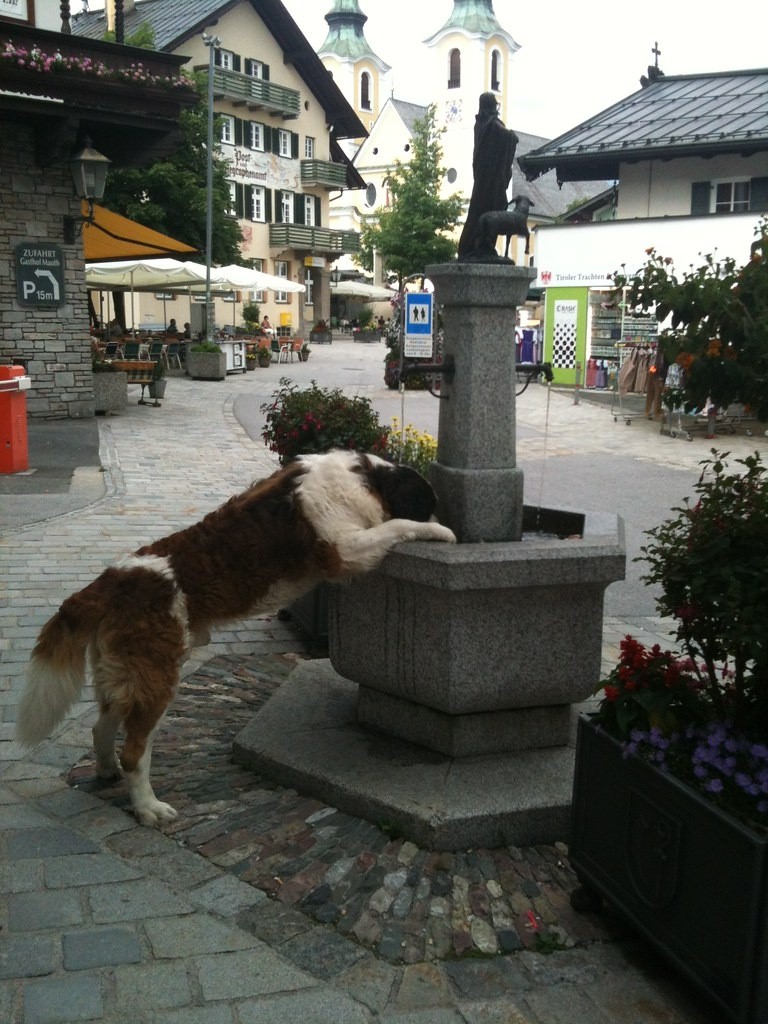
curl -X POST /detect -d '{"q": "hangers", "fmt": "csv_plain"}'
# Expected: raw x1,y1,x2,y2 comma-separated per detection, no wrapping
627,340,658,357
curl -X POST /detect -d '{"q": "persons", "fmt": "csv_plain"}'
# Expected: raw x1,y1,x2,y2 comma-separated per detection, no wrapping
184,323,191,336
166,318,179,335
331,315,391,333
261,316,277,339
93,321,102,335
90,325,104,362
105,318,122,341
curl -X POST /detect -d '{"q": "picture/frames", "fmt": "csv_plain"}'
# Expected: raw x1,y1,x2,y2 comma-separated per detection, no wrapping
566,709,768,1024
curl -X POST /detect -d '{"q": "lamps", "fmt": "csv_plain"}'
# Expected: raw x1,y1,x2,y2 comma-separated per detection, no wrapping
329,266,341,287
62,143,113,246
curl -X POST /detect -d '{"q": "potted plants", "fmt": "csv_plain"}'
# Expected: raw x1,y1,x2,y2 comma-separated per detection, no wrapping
297,343,311,361
187,340,230,378
147,366,167,398
257,347,272,368
309,317,333,344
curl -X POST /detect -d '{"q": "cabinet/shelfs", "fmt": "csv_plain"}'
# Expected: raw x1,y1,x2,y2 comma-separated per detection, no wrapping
217,340,254,373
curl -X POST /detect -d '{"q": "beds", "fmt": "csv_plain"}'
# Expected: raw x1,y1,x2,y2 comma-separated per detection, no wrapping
162,343,184,371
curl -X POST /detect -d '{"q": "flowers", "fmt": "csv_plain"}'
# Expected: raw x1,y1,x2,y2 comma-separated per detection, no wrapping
245,354,256,370
353,321,379,332
599,631,768,838
262,375,388,468
385,416,439,477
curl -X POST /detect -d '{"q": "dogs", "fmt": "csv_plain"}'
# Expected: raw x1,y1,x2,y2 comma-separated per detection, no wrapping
14,447,457,828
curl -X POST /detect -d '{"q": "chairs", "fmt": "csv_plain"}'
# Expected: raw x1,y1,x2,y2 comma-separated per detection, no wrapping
119,341,141,359
258,336,304,364
104,342,119,358
144,343,164,360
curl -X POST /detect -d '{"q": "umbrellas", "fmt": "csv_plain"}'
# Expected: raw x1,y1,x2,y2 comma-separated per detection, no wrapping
330,280,399,322
85,258,306,342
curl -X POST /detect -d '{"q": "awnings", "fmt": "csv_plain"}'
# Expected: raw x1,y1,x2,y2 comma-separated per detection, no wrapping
81,198,201,264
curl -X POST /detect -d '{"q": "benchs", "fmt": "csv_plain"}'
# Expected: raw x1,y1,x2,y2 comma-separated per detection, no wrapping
110,361,161,406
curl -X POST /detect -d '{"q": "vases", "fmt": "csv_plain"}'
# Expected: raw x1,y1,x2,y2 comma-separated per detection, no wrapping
354,332,381,344
